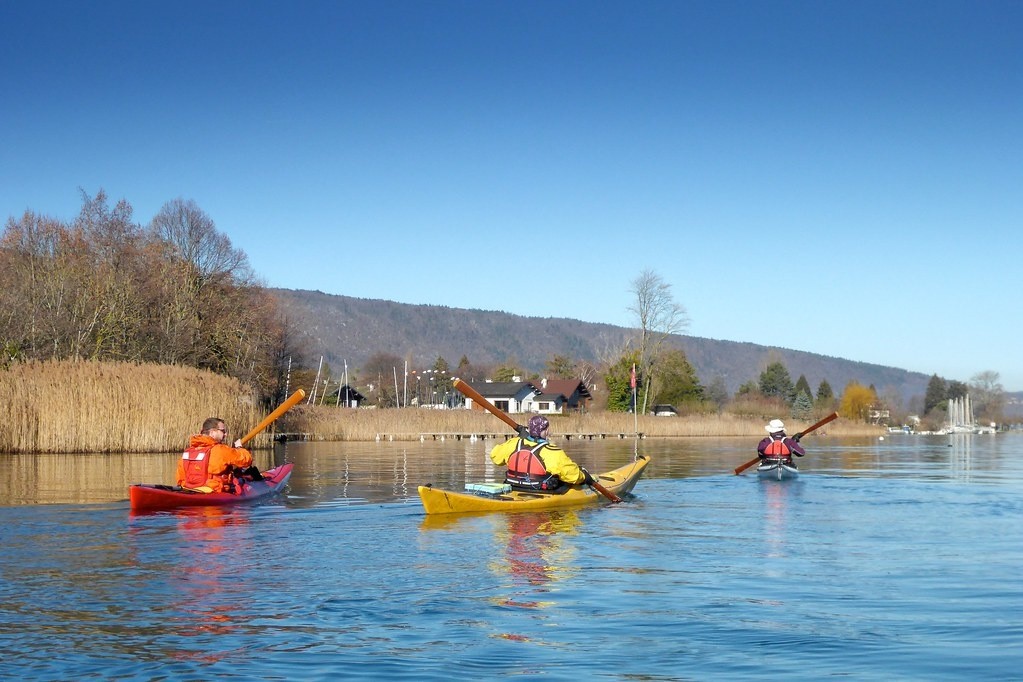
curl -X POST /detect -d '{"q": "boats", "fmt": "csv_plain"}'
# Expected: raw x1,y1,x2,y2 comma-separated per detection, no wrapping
130,462,296,510
416,454,651,515
757,455,800,479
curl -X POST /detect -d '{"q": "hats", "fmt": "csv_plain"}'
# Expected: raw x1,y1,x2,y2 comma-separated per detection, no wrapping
530,416,549,437
765,419,787,433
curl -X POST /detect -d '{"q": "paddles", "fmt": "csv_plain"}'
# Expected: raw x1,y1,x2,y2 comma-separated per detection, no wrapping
734,411,839,476
242,387,306,446
452,377,621,503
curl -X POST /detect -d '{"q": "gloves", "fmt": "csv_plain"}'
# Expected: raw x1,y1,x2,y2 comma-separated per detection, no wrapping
579,466,590,486
517,427,529,439
792,433,803,442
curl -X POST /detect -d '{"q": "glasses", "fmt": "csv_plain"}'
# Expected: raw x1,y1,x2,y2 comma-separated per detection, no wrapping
212,428,226,434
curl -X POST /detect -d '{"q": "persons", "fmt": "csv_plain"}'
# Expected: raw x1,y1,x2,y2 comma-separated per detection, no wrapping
490,415,599,491
175,417,253,492
758,419,805,465
